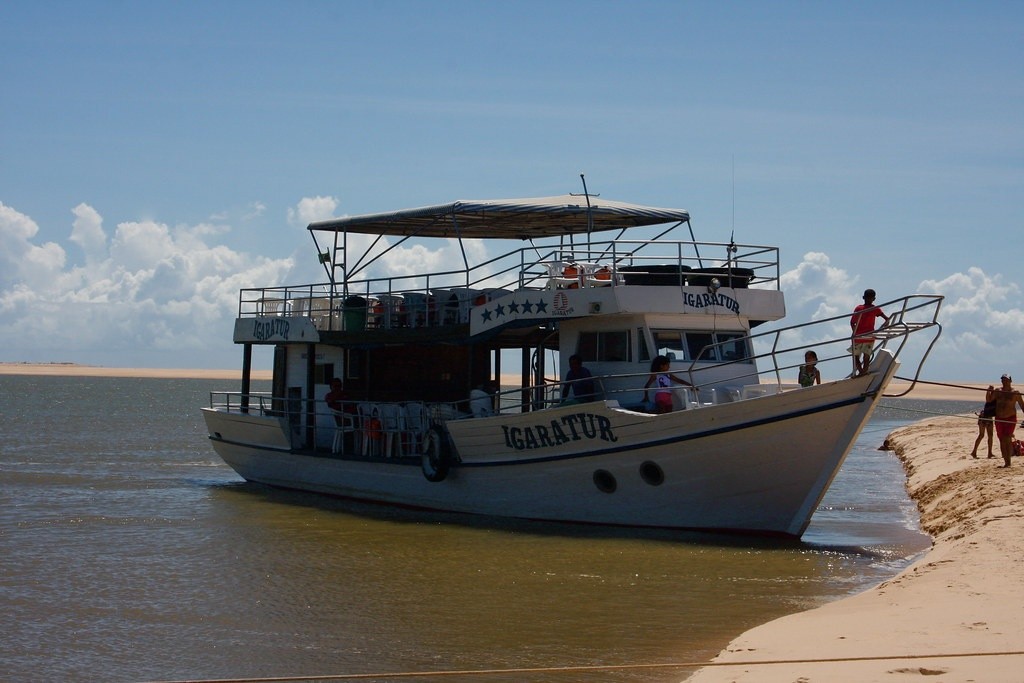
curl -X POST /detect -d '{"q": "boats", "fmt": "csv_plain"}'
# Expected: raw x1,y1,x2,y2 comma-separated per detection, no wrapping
200,170,945,538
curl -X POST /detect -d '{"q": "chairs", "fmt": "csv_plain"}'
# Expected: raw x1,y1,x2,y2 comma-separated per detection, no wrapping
541,260,633,290
328,400,454,457
256,288,514,331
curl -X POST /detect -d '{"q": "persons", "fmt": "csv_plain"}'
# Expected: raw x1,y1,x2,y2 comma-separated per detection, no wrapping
985,374,1024,468
847,289,890,377
798,351,820,389
640,355,700,414
469,380,498,418
971,399,997,459
325,377,358,455
556,355,597,408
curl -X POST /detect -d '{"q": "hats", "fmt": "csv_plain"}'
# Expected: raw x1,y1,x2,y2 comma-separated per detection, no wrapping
1001,374,1011,380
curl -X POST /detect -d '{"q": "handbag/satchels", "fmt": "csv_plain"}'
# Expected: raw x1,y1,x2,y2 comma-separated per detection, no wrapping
984,392,996,418
1012,440,1024,456
